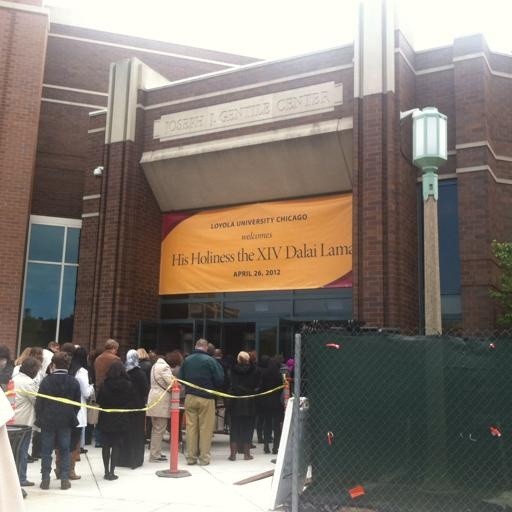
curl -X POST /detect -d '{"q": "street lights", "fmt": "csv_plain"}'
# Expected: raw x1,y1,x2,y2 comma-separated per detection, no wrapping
400,108,452,334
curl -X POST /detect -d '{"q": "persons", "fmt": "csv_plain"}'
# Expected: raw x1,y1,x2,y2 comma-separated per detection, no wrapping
0,337,292,510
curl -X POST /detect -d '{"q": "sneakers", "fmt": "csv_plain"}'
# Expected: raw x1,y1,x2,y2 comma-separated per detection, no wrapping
251,444,255,447
62,480,71,489
27,458,33,463
146,439,151,442
188,461,197,465
110,474,118,480
70,473,81,480
95,444,101,447
199,461,209,465
40,480,50,489
20,480,34,486
104,474,110,479
150,459,168,464
80,448,88,454
28,456,38,461
160,455,166,458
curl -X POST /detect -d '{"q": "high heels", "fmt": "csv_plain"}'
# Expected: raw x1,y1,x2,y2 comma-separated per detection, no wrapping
264,448,270,454
272,448,277,453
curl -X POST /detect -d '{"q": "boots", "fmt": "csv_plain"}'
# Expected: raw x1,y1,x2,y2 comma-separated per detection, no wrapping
229,441,237,461
244,443,253,460
